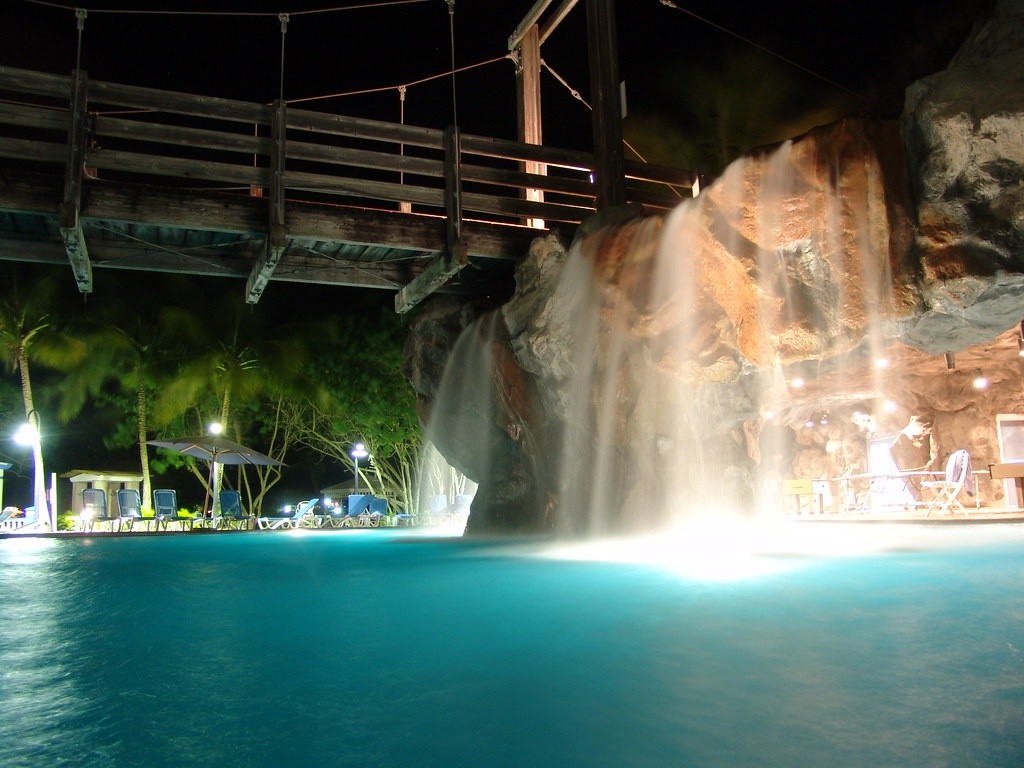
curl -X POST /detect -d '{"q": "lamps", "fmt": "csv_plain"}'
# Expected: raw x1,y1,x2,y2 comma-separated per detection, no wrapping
944,353,956,370
1018,338,1024,356
805,418,813,427
821,417,828,424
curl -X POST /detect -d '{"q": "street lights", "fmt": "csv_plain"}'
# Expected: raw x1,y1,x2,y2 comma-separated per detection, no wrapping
17,411,41,533
352,443,369,495
210,424,222,530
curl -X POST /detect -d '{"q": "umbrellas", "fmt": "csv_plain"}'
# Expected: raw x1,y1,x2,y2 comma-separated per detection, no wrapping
138,436,289,528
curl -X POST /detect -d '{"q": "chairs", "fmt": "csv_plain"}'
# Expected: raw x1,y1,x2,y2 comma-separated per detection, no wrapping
81,489,122,532
0,507,38,533
116,488,156,532
152,489,193,531
921,450,970,518
220,490,256,531
258,494,473,531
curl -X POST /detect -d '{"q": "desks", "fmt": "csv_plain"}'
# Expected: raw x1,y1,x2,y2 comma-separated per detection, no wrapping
832,470,990,511
